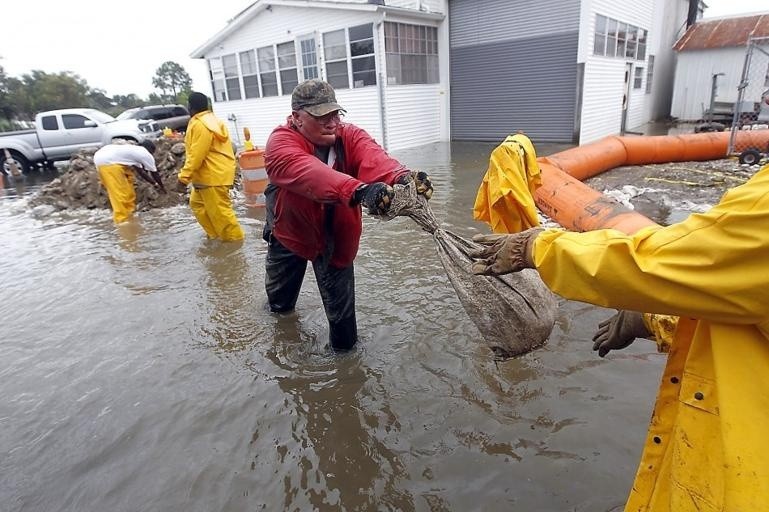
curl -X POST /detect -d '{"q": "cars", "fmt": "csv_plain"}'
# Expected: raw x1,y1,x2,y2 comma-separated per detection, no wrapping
117,104,190,134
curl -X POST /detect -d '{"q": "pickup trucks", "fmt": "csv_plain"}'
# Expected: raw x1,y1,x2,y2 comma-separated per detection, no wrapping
0,108,163,177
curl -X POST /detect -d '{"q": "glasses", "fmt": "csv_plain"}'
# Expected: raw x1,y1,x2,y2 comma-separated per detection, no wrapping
314,113,339,124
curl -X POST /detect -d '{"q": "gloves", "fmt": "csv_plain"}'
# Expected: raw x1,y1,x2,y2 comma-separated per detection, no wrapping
592,310,648,358
469,227,545,275
399,171,433,199
176,179,187,194
356,182,395,214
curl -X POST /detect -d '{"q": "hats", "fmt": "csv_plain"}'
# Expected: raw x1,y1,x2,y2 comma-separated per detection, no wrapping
188,92,208,108
292,79,347,116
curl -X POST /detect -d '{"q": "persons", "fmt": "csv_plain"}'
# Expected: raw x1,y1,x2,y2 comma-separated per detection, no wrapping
176,92,244,241
470,163,769,512
264,79,432,352
93,139,167,223
